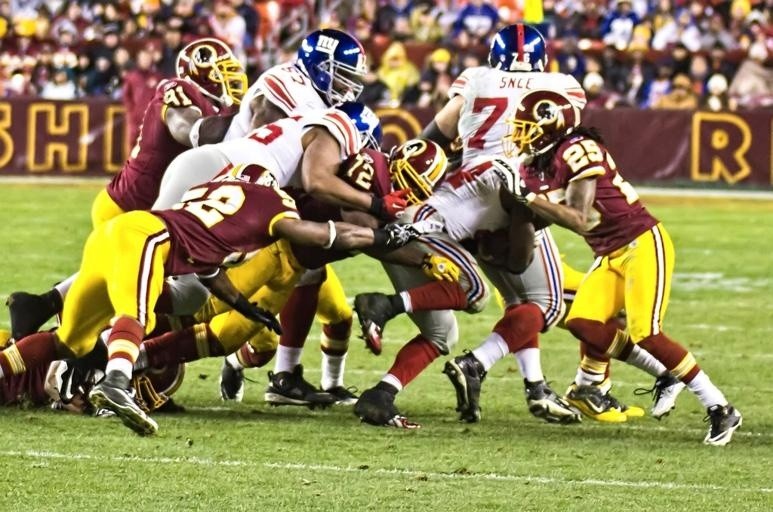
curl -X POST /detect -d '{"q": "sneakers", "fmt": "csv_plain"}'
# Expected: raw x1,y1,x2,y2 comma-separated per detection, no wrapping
442,348,489,425
5,292,51,341
699,405,743,446
88,382,158,436
633,375,687,420
42,359,88,404
522,378,643,424
264,364,420,429
219,357,245,404
354,292,395,355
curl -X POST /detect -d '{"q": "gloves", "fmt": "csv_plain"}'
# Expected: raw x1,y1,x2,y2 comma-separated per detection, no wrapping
371,189,412,221
492,158,537,206
255,302,283,336
380,222,410,249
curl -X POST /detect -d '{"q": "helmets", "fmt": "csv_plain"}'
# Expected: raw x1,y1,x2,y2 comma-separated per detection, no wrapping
487,24,549,71
176,36,249,105
389,138,449,207
504,91,575,160
88,361,184,418
294,29,367,109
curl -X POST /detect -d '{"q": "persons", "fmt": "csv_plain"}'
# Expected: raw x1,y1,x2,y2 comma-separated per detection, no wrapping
1,27,742,446
1,0,773,112
